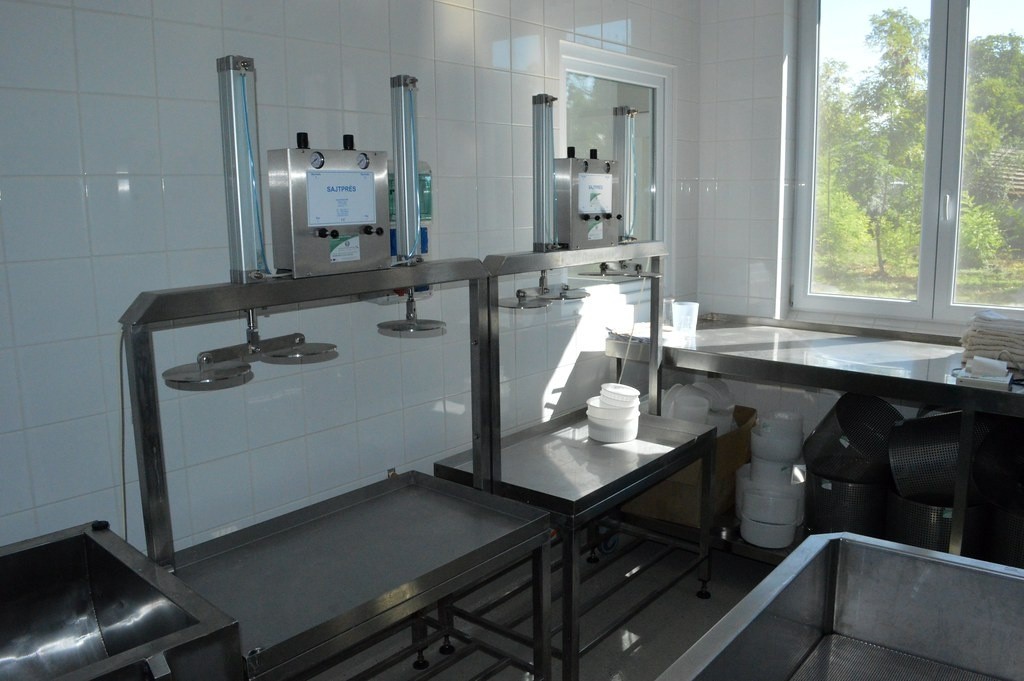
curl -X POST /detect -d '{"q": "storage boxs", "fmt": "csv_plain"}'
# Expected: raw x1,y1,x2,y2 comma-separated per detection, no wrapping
617,402,761,529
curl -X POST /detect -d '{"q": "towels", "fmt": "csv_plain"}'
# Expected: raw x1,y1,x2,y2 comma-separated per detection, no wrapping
959,309,1023,369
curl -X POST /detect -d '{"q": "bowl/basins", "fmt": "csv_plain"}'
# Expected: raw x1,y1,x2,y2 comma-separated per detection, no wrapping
736,413,804,548
640,379,730,424
586,382,641,442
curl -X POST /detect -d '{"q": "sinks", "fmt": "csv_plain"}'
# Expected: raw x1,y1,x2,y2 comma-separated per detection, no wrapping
650,533,1022,679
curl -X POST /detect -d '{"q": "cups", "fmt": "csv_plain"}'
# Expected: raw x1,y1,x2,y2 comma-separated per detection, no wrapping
672,302,699,338
663,298,674,331
